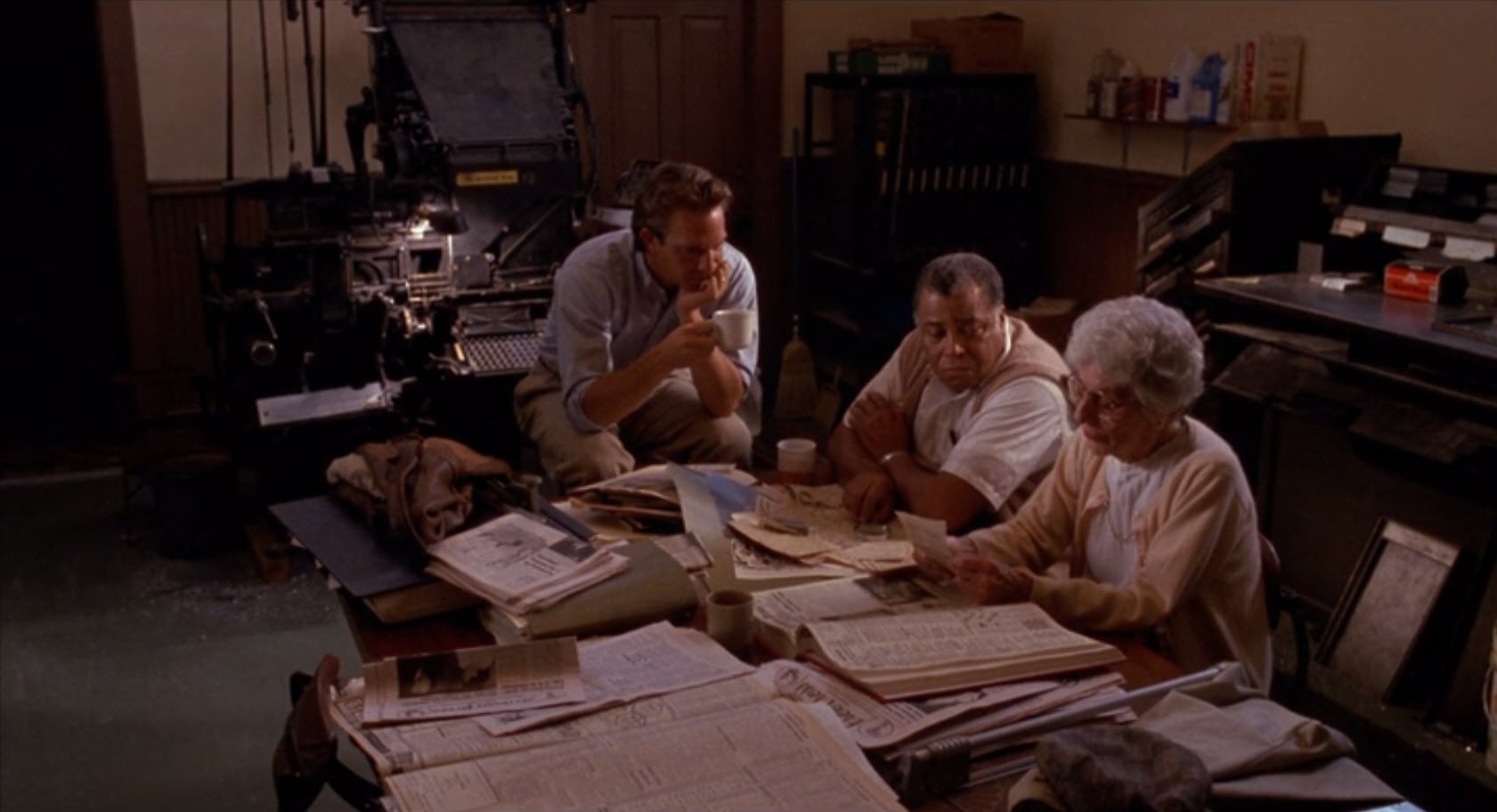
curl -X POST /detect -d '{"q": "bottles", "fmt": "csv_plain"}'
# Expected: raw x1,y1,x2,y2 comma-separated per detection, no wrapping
1085,47,1225,122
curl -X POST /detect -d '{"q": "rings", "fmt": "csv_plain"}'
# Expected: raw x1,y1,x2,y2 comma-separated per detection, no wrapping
717,277,725,282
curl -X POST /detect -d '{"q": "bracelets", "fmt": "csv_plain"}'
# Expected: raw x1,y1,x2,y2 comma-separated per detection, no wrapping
882,450,910,464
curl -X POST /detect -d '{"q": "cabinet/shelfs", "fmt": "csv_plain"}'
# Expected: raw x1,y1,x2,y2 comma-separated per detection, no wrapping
790,70,1043,397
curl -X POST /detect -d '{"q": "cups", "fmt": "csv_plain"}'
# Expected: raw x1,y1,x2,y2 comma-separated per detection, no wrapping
712,309,754,350
777,438,817,472
706,590,755,655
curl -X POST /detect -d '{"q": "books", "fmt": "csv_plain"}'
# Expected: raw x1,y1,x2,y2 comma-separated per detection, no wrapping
747,565,1127,704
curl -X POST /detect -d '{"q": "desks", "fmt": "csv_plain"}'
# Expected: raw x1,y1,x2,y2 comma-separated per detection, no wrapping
1187,274,1497,747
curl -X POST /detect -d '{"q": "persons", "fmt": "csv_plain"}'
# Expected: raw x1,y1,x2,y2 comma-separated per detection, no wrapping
915,298,1274,700
827,253,1088,536
513,162,758,498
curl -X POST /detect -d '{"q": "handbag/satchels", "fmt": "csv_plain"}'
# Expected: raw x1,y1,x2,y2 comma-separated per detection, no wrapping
327,440,517,543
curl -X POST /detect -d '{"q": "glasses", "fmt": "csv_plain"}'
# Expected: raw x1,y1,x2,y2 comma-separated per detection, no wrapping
1067,372,1152,422
